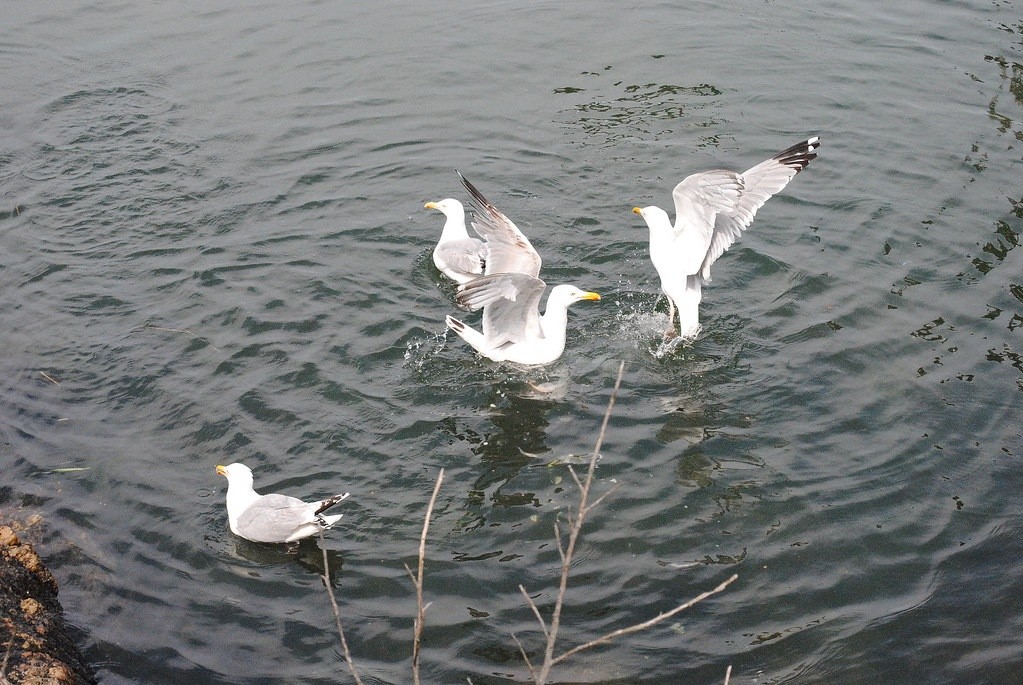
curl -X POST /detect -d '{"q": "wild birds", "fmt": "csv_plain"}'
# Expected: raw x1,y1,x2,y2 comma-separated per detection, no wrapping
444,169,602,366
425,197,487,286
215,462,350,544
632,136,822,344
